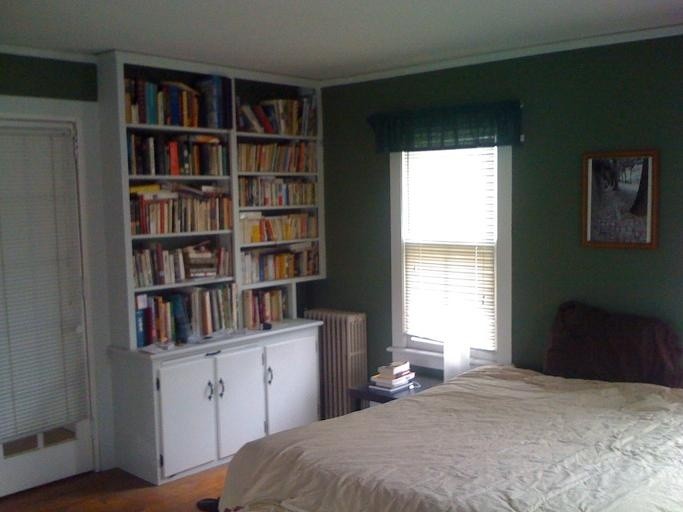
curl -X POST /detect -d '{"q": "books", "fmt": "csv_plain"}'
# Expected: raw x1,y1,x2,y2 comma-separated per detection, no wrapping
368,361,416,393
126,78,233,345
237,94,320,284
237,287,286,331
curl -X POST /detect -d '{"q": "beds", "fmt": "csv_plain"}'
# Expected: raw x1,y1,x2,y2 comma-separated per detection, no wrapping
217,362,683,510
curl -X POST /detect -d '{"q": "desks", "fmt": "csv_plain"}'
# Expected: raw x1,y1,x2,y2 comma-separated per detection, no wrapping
347,375,442,414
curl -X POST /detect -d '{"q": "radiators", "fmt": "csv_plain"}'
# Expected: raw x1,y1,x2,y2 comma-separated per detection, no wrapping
305,308,367,418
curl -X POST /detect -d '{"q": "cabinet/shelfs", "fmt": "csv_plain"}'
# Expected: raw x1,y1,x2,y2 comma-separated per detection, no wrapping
104,344,268,481
264,335,320,439
97,49,328,350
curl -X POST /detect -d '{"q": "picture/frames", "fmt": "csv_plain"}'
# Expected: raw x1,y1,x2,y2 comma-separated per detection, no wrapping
580,148,657,251
